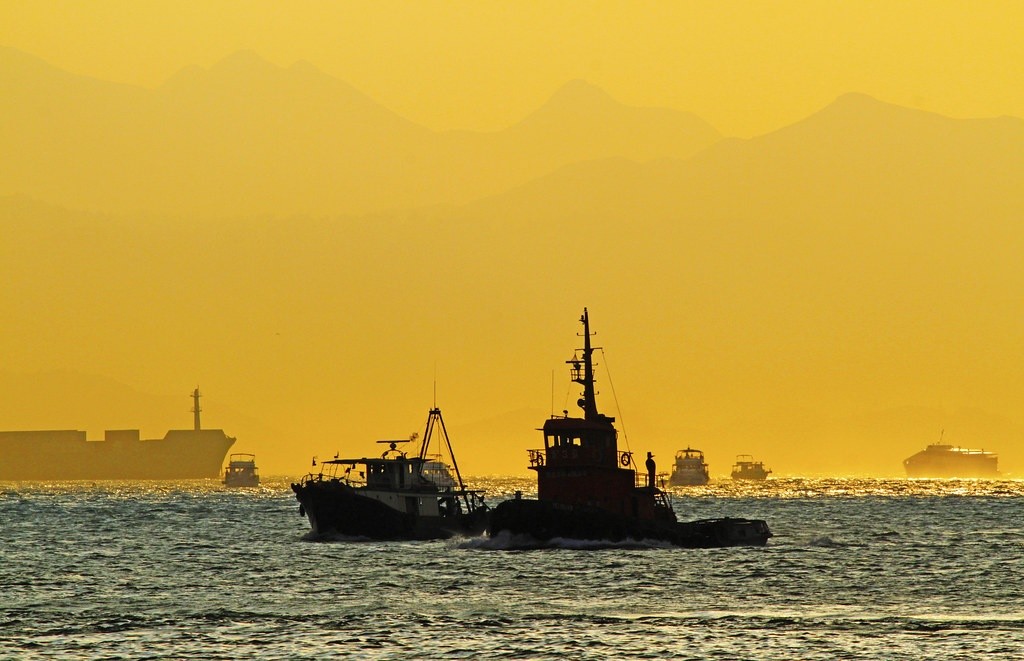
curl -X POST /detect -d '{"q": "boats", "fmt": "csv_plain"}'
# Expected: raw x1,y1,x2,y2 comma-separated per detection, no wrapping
0,385,237,478
903,430,1000,479
671,448,711,488
289,380,491,540
222,454,262,487
730,456,773,484
489,307,773,548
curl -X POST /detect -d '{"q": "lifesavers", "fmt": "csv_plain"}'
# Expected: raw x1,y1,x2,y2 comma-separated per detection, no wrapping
537,454,544,465
299,503,305,516
622,453,630,465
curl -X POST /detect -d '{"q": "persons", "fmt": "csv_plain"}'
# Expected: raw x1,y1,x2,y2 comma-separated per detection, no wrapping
645,452,656,486
454,496,462,514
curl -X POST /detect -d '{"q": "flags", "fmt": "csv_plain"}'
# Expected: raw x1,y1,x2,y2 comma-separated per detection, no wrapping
571,355,581,370
